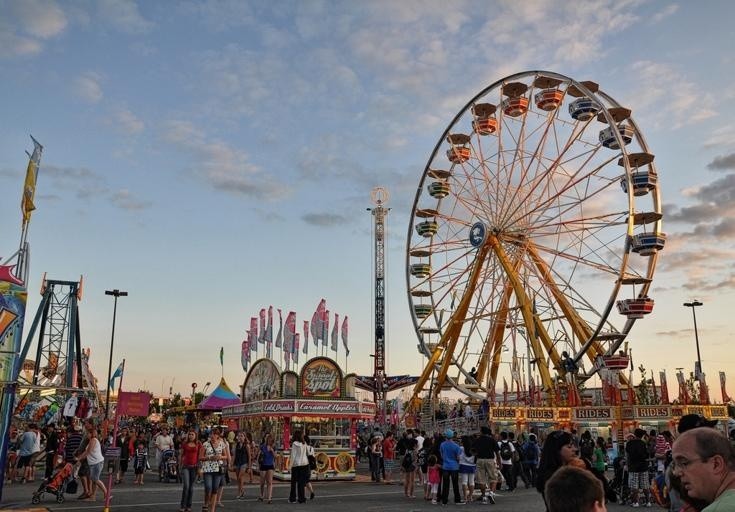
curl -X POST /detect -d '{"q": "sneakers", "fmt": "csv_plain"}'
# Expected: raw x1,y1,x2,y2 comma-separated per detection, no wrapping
462,496,473,502
629,502,640,508
77,492,113,503
487,492,496,505
177,490,315,512
641,502,652,508
479,496,487,505
505,479,538,493
404,492,447,507
455,500,467,505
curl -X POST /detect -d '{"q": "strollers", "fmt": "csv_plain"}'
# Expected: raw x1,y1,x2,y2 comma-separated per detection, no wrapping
159,450,182,484
32,459,83,504
609,456,658,507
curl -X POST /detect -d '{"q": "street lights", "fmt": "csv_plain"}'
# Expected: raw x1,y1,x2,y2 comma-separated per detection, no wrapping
104,288,128,421
683,298,704,373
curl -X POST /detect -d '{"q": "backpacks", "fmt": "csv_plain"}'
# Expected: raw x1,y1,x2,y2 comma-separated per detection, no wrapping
498,440,513,460
525,444,536,461
648,469,671,509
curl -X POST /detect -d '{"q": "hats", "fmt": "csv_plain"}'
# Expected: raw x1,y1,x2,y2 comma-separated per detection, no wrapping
678,414,719,433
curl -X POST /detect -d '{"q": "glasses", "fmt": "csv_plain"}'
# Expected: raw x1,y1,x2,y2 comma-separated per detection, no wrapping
672,456,711,471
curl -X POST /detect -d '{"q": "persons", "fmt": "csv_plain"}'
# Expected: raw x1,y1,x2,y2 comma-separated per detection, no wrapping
5,411,735,511
